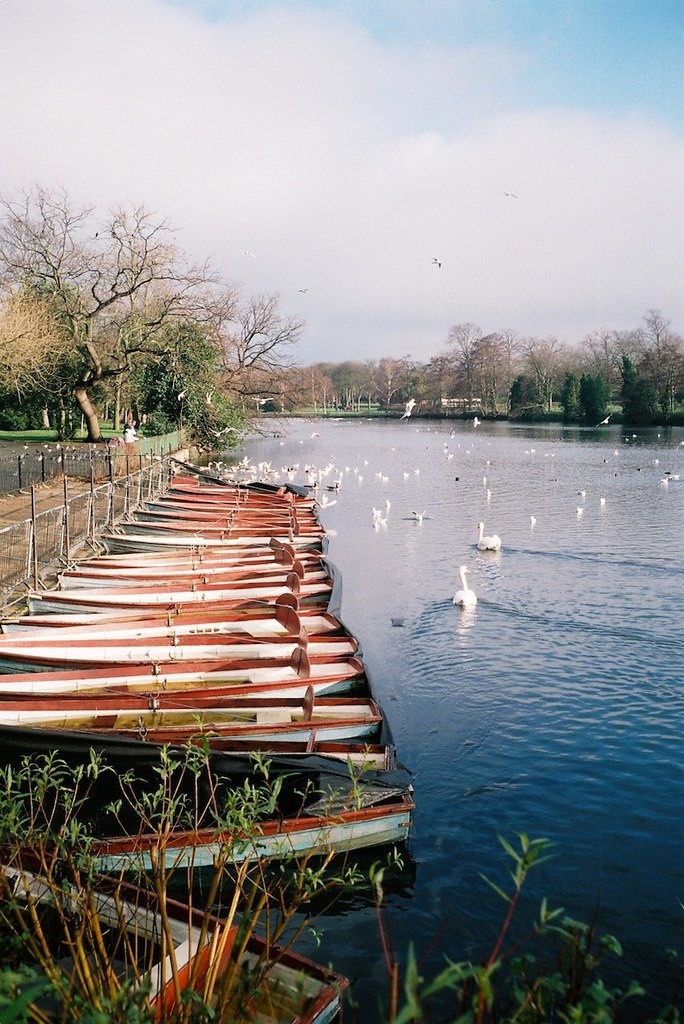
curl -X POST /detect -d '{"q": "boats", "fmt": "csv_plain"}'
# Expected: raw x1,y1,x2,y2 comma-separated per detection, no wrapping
0,477,417,875
0,847,350,1024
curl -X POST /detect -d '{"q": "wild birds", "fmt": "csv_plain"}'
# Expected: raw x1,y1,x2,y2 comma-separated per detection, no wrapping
0,399,683,526
297,288,308,294
503,192,520,199
431,257,442,268
239,249,256,258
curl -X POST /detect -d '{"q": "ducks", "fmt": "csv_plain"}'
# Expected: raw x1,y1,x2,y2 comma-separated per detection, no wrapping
477,522,501,551
453,566,477,607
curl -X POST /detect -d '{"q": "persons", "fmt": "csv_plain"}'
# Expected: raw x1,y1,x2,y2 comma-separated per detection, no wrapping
123,410,147,443
